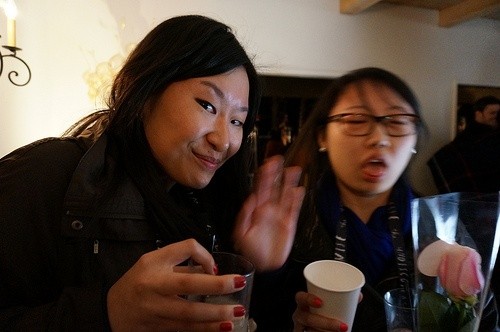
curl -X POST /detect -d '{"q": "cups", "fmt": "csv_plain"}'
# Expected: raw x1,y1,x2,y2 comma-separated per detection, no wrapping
303,259,366,332
185,252,256,332
383,290,416,332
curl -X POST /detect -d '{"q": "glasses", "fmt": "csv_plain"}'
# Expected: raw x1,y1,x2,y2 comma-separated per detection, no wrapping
325,113,421,137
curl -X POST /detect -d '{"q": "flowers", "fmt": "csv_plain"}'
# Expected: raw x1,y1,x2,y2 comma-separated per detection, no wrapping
415,237,485,332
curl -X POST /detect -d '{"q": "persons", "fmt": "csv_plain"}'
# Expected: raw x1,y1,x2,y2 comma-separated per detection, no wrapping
431,95,500,298
247,67,477,332
0,14,306,331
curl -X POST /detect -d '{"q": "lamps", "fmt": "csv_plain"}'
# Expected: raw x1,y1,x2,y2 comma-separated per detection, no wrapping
0,1,32,86
